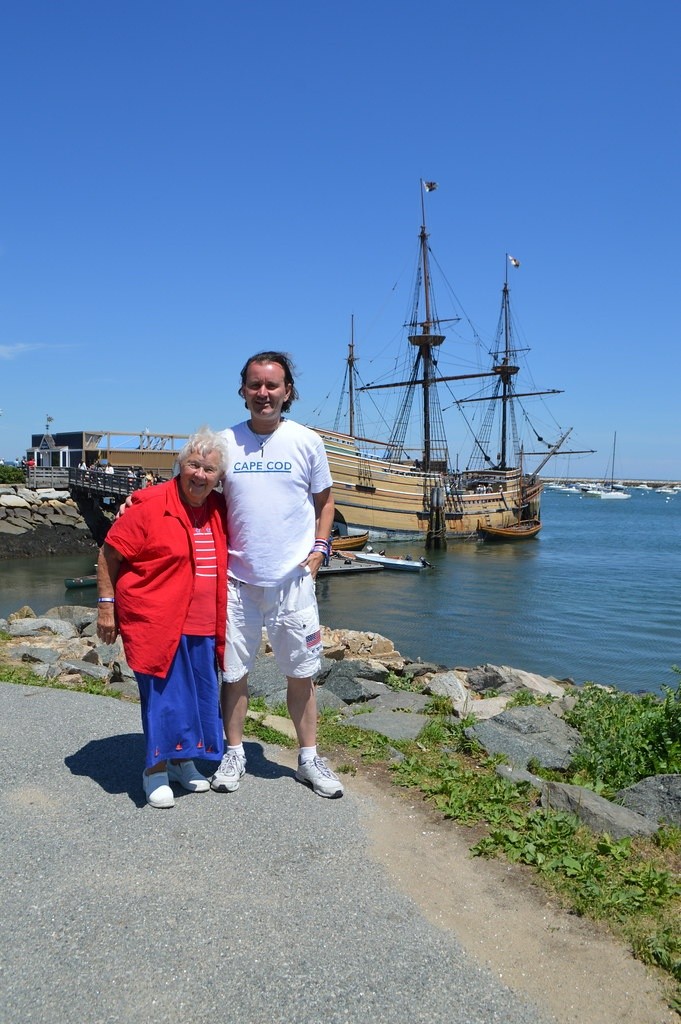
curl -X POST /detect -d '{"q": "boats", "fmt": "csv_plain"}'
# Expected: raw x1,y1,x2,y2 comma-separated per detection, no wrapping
542,479,681,501
316,561,387,575
355,551,433,572
328,529,372,551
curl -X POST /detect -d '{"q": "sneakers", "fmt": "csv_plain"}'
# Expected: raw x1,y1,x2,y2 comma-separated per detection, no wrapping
142,768,174,808
294,754,343,798
209,750,246,792
166,760,209,792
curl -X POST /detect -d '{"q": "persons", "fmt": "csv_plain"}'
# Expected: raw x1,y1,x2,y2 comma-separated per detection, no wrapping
0,455,35,472
96,423,229,808
438,471,505,502
116,352,345,799
77,457,167,491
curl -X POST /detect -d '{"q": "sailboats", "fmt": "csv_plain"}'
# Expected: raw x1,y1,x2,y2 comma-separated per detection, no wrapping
479,441,544,539
288,171,597,542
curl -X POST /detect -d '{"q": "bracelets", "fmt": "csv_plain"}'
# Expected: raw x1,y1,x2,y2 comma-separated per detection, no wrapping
311,536,330,557
98,597,115,603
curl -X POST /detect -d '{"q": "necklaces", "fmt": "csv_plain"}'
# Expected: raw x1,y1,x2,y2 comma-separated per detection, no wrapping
249,418,284,458
